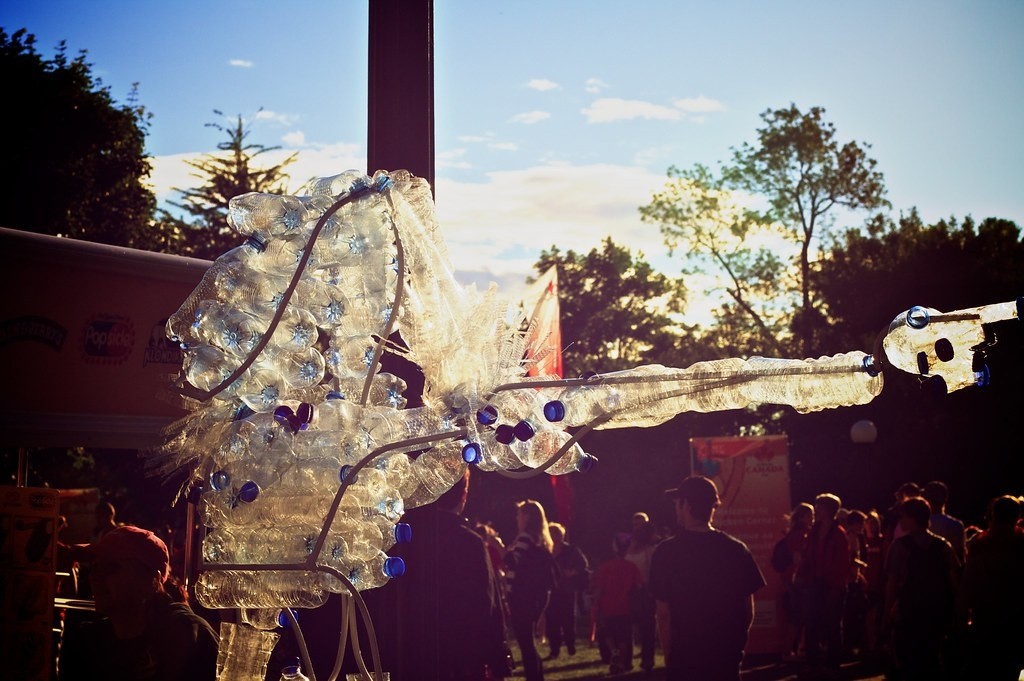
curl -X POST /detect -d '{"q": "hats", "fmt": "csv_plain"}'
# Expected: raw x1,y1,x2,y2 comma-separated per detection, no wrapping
898,482,925,495
73,526,169,584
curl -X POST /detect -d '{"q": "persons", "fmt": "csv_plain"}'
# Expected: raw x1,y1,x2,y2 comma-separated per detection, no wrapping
56,501,375,681
472,500,669,681
769,482,1024,681
652,476,767,681
375,468,512,681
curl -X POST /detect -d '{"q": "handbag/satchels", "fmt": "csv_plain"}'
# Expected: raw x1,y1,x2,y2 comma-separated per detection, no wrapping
771,540,796,569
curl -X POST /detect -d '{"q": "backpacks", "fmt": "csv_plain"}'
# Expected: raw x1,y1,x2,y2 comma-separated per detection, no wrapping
531,542,566,598
897,535,958,623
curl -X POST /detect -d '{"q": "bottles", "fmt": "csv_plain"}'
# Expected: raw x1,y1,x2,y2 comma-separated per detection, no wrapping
170,170,992,681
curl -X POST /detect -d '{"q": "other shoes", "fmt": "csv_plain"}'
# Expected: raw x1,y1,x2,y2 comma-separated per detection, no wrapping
566,641,575,656
823,662,840,675
634,652,644,658
640,663,651,671
549,649,560,659
623,664,634,671
783,655,798,662
